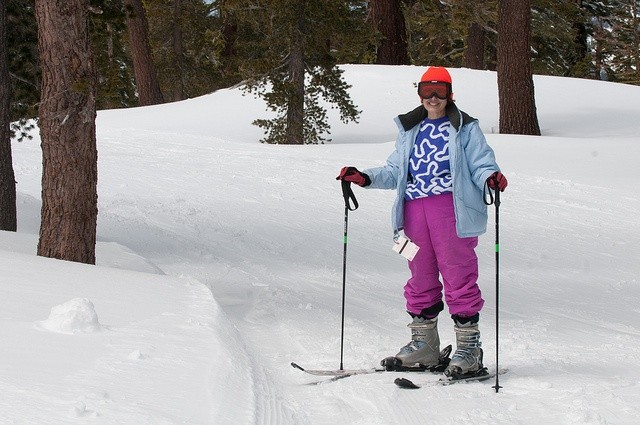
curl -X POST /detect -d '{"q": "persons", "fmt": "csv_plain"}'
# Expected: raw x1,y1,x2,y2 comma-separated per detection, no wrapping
335,65,509,377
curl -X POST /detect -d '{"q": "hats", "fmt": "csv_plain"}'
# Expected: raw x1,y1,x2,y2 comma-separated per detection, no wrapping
418,67,454,100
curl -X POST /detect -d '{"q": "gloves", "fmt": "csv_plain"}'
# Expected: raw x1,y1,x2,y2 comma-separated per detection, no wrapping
489,172,508,192
336,167,365,186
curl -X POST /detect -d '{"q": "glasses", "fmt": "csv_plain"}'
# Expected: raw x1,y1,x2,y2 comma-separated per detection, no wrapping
418,82,449,99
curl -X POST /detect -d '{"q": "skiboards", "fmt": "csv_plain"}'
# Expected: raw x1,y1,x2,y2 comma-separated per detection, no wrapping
291,362,509,388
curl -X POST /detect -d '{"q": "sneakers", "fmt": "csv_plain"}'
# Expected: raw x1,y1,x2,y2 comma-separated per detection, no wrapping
448,319,482,374
395,316,440,368
